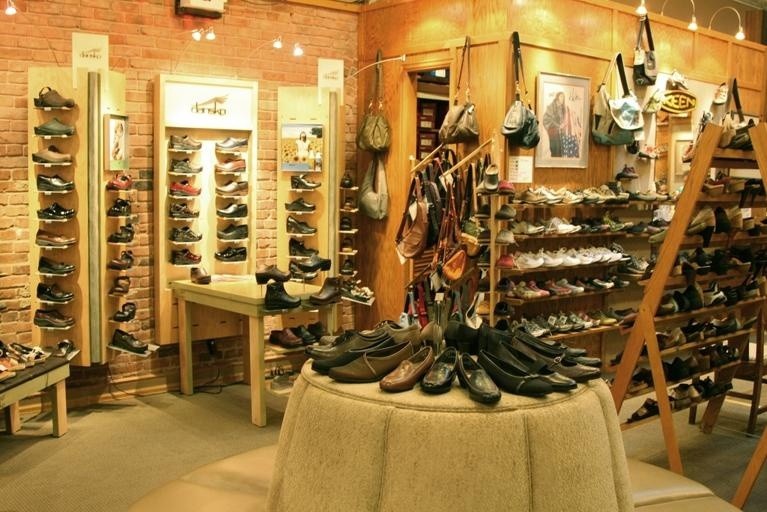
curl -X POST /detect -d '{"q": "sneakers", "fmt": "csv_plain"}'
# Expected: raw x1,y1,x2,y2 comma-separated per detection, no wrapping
571,214,634,234
605,275,630,287
534,218,558,236
578,247,603,264
577,311,601,326
598,247,623,263
616,165,639,181
682,111,713,163
567,311,593,329
515,281,541,300
719,118,757,151
545,280,572,296
600,181,631,205
516,253,544,269
609,243,631,262
574,188,599,205
532,321,550,334
545,313,573,332
546,250,581,267
565,247,593,265
550,186,584,205
530,251,564,268
528,186,563,205
541,216,581,234
527,280,550,298
521,318,543,338
593,278,615,289
556,278,584,294
536,280,556,296
558,314,585,331
509,187,547,204
531,315,555,331
548,217,575,234
594,247,611,263
509,220,545,235
576,280,607,292
638,146,660,160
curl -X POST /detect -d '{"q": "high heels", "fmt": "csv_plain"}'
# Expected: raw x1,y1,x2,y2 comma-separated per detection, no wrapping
7,343,46,363
702,177,724,197
750,179,762,206
0,371,10,382
287,216,317,234
291,173,321,189
739,178,756,208
420,321,443,347
3,348,35,368
726,205,743,255
13,343,48,358
0,352,25,371
715,206,732,255
715,171,745,192
0,365,17,378
289,238,319,256
648,204,716,249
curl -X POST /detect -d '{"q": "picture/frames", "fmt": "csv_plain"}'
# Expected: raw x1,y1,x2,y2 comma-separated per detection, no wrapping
104,113,129,172
280,123,324,175
533,71,592,170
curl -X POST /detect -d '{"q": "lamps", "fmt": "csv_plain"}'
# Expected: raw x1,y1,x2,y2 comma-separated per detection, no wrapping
657,5,697,31
343,48,412,87
166,28,217,78
6,0,65,65
234,35,285,76
706,2,747,45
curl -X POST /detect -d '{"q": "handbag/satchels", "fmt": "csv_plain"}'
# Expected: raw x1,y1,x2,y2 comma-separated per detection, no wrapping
393,148,456,259
632,13,657,86
357,152,388,220
500,31,541,150
591,52,645,146
437,35,480,144
398,153,491,329
356,49,391,152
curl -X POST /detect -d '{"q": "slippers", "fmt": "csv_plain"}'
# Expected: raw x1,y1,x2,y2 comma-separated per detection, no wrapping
494,204,517,220
497,276,510,291
495,256,514,269
32,145,72,163
285,198,315,211
495,229,515,245
34,118,75,136
498,179,515,194
298,253,331,272
255,265,292,284
493,301,508,316
483,163,499,193
33,85,75,108
494,319,509,331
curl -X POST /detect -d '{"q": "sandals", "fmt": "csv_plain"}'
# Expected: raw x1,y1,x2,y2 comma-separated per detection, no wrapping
619,280,767,326
610,318,757,366
51,339,73,358
661,344,740,382
610,378,649,394
638,244,767,280
665,376,734,410
339,277,374,302
631,398,672,421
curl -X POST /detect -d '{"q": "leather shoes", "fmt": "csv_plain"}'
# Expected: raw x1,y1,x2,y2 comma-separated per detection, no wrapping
308,321,330,339
214,247,247,262
170,158,203,174
343,196,354,210
477,349,554,397
341,237,353,252
269,327,303,348
421,345,459,393
215,137,249,153
169,180,201,196
171,226,202,242
340,259,354,275
36,283,74,302
380,345,434,392
289,261,317,280
446,311,463,344
109,250,134,270
319,320,422,351
339,217,352,231
215,180,249,197
496,340,577,391
216,224,249,240
627,190,656,201
303,329,389,360
618,254,654,274
309,276,341,306
36,203,75,219
311,336,396,374
631,369,655,387
171,249,201,265
215,158,246,173
461,319,482,354
108,223,134,243
458,352,500,403
519,333,603,368
604,309,623,321
290,325,317,344
113,302,136,323
111,275,131,296
265,282,301,311
586,310,617,326
216,203,248,218
112,329,149,353
644,191,668,201
516,325,588,357
169,203,199,218
34,309,74,328
626,216,670,235
476,318,513,343
37,255,76,275
328,340,413,383
35,229,77,247
168,135,202,151
36,173,75,192
190,267,211,285
341,174,353,188
108,197,132,217
511,336,601,383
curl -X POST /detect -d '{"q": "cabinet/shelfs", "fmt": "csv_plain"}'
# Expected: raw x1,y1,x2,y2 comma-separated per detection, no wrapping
159,77,262,336
610,123,767,476
277,85,359,274
497,142,742,379
29,66,135,365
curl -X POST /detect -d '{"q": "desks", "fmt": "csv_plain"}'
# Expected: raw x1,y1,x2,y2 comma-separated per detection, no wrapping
171,273,344,429
0,346,71,438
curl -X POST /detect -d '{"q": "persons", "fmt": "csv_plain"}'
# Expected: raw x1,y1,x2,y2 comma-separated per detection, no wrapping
543,91,573,157
295,131,310,155
112,122,124,161
